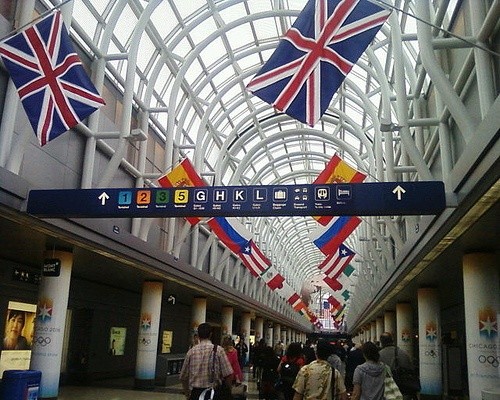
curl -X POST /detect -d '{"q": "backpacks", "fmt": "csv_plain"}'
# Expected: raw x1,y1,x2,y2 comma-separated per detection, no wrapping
280,355,300,394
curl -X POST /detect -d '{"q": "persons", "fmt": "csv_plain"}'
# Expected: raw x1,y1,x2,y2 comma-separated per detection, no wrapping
3,310,27,350
178,322,422,400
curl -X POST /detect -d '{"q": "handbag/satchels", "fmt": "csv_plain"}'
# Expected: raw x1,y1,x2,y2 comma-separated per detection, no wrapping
399,368,421,392
384,367,403,400
272,373,279,388
204,387,231,400
230,383,247,399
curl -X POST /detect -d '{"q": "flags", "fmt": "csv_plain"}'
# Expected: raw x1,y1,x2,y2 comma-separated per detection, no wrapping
318,243,356,281
156,157,209,226
312,216,363,258
243,0,394,128
310,152,368,228
205,217,256,258
0,8,106,147
238,240,271,279
260,264,356,330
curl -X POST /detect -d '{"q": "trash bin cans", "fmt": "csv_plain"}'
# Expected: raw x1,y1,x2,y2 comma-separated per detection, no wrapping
2,369,42,400
155,353,187,388
